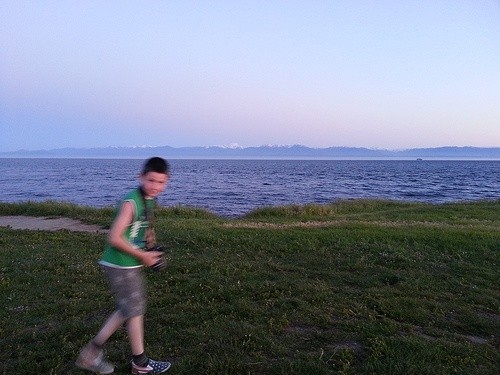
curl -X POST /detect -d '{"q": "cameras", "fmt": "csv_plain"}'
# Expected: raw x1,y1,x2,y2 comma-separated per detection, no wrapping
146,244,167,272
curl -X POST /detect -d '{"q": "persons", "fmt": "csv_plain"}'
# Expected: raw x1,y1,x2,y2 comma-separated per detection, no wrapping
76,156,172,375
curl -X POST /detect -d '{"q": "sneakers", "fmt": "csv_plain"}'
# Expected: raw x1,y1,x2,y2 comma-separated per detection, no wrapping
131,357,171,375
76,357,114,375
